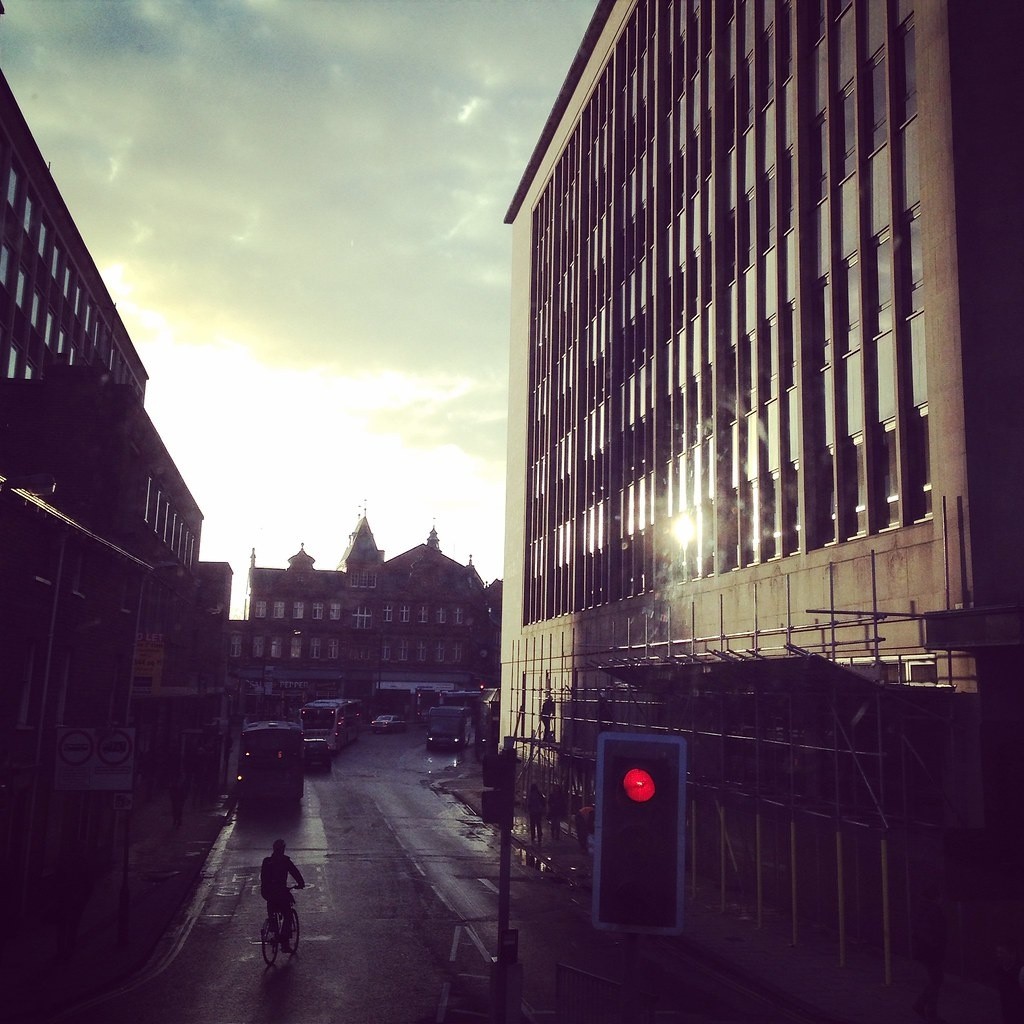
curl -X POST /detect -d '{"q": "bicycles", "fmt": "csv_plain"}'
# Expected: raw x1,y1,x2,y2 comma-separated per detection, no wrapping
260,884,303,965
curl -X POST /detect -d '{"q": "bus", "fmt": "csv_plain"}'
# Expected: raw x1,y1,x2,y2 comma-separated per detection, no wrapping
425,705,468,751
297,698,364,750
236,718,307,802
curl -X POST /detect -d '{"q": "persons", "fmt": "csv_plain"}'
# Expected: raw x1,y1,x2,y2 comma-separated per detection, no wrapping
169,725,233,827
527,785,567,843
574,808,595,852
540,696,555,742
261,839,305,954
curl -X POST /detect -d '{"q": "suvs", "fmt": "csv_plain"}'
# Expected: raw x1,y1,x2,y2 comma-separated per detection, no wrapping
303,736,334,770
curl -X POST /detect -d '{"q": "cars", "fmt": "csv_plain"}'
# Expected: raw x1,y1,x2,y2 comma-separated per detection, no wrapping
371,714,407,733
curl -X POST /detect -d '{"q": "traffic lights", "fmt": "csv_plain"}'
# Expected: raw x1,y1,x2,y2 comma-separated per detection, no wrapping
593,729,687,938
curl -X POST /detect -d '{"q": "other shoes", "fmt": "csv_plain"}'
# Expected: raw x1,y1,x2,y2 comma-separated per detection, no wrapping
191,801,221,813
173,818,182,826
531,836,543,843
281,934,293,940
551,832,560,837
